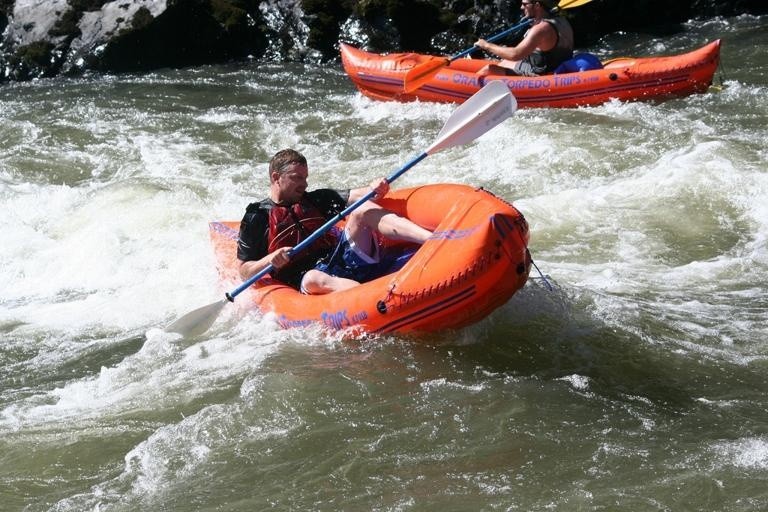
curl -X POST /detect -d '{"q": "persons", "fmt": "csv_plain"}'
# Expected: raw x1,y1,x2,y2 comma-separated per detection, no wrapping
474,0,574,76
237,148,434,296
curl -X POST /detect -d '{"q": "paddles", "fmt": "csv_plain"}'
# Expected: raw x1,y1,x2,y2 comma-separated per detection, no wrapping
163,79,518,336
404,1,589,94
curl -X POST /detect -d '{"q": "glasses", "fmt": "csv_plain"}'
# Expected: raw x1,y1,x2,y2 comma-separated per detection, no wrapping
522,3,533,6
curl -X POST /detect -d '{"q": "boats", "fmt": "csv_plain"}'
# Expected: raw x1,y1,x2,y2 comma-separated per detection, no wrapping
207,182,533,341
337,38,723,107
